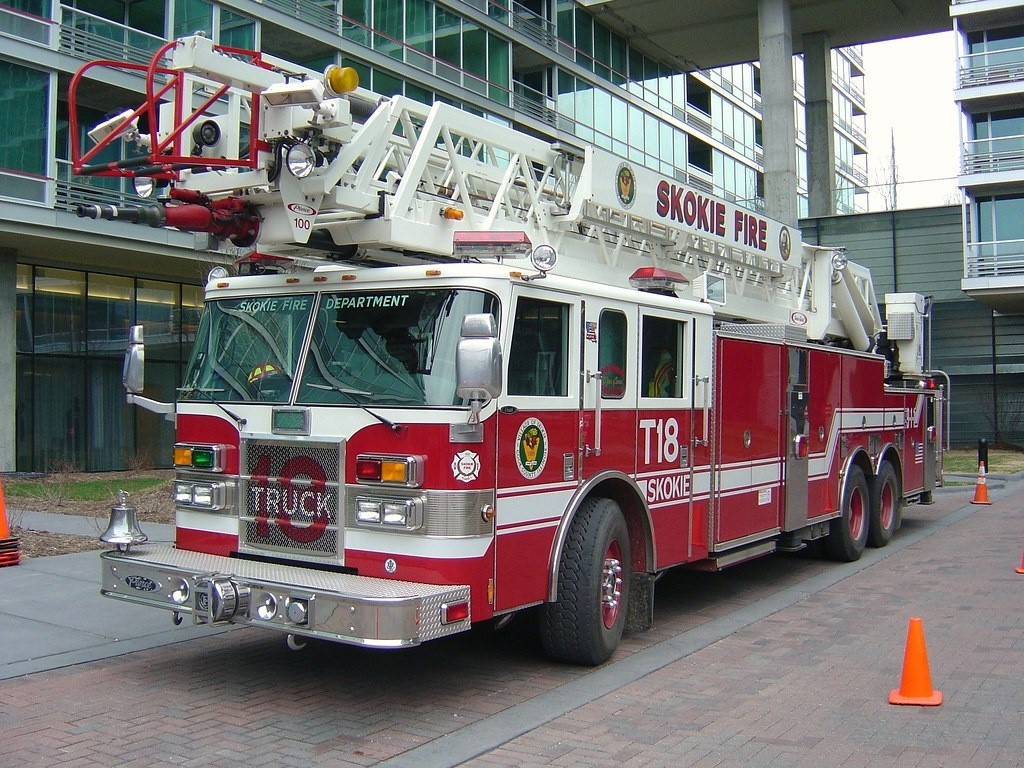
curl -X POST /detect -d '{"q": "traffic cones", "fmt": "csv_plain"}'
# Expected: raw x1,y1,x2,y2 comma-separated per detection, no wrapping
0,477,10,541
1015,549,1024,573
970,461,993,505
889,618,943,706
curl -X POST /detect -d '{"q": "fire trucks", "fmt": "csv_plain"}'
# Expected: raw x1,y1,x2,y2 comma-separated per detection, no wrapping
68,32,936,667
